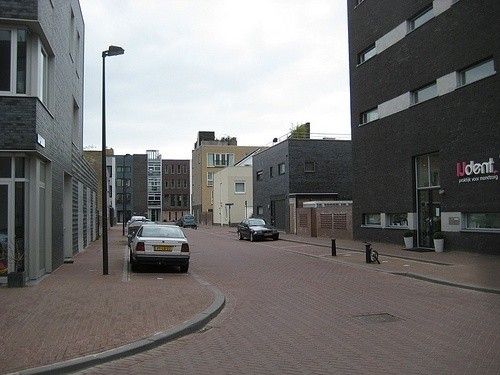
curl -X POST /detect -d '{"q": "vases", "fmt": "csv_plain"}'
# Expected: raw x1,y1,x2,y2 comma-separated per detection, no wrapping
8,271,25,287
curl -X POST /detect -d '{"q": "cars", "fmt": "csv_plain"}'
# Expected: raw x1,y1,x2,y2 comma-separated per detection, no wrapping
126,215,154,243
178,214,198,228
236,218,280,242
128,224,191,273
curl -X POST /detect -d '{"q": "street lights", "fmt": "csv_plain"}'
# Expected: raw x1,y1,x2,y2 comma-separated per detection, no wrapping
101,44,128,275
122,153,130,236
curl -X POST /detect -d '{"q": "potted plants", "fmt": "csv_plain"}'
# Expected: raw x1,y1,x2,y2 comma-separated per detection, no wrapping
433,232,445,252
402,231,414,248
